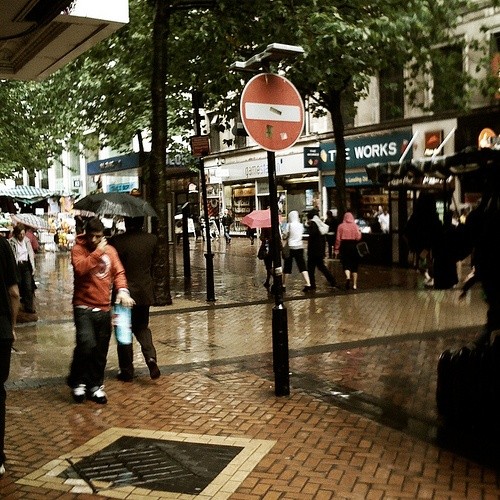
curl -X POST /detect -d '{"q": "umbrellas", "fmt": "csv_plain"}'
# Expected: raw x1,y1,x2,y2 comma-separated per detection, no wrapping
240,209,284,230
72,192,159,217
10,212,52,230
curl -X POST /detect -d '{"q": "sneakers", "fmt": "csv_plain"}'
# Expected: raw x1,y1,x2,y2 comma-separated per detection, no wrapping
71,384,108,404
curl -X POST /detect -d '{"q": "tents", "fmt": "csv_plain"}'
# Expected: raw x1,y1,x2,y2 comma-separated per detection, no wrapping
0,184,78,216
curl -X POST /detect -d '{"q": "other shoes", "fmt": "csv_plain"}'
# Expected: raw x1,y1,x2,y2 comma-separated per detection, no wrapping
117,358,161,382
263,277,358,292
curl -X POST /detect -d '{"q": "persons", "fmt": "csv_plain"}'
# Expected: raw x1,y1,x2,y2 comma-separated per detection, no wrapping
66,215,137,403
367,206,390,235
7,226,39,314
0,235,22,476
324,211,337,259
208,215,220,242
221,209,234,243
259,227,283,287
281,211,313,293
246,226,256,245
304,208,337,290
107,217,161,381
53,230,61,253
335,212,362,290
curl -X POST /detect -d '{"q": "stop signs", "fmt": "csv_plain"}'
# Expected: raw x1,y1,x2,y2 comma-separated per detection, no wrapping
237,71,308,150
